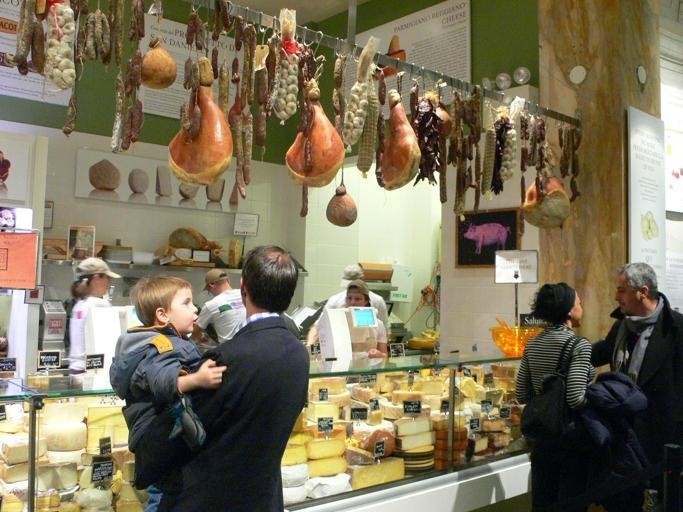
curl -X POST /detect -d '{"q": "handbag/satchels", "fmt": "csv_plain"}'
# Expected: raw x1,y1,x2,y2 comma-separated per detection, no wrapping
520,334,590,441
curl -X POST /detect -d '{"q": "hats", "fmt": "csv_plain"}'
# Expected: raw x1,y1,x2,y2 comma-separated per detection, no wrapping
341,264,363,287
198,268,231,293
73,256,122,280
346,280,370,295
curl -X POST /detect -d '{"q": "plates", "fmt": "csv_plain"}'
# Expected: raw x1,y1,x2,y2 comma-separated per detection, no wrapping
392,447,437,471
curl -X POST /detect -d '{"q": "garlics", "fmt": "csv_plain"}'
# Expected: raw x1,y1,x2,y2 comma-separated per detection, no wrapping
499,129,518,182
43,2,77,85
342,82,369,145
275,54,299,117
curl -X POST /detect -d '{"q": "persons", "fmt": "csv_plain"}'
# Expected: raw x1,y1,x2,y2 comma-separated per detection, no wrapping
589,263,681,511
68,254,118,372
517,284,597,511
338,278,387,360
129,242,311,511
192,261,250,344
110,277,227,512
306,264,388,347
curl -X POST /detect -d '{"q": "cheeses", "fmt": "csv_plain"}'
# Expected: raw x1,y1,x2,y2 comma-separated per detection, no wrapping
0,398,146,512
281,365,521,491
156,166,174,198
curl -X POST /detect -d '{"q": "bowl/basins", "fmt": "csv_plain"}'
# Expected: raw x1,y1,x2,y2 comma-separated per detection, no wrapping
489,326,547,355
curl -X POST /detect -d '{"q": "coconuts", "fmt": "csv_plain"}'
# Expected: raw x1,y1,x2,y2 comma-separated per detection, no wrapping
89,159,121,191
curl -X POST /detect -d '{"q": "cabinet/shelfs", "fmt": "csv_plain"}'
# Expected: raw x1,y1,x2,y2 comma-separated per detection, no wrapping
0,351,531,512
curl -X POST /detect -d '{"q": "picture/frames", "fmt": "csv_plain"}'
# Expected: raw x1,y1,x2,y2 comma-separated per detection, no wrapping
66,225,96,262
454,207,524,269
191,249,211,264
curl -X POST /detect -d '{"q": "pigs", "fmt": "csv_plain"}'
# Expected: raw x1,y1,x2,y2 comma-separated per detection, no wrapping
464,223,512,255
